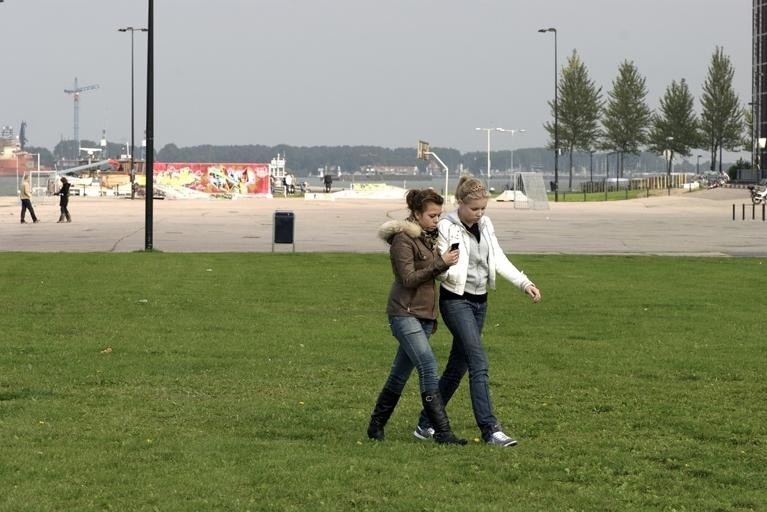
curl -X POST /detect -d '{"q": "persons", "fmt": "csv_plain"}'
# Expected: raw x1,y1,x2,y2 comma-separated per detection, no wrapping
412,174,542,450
283,172,332,198
365,187,470,447
19,173,40,225
53,177,73,223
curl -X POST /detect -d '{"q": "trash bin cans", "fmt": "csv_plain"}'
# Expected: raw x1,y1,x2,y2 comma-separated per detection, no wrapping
272,209,295,244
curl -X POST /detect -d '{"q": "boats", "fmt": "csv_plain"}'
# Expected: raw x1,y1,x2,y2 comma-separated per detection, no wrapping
0,124,210,199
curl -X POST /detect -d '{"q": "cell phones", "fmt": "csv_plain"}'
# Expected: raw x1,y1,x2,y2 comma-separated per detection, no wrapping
451,242,459,250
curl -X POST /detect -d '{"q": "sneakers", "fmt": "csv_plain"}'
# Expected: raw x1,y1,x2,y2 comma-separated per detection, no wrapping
412,425,435,440
486,429,517,447
21,220,40,224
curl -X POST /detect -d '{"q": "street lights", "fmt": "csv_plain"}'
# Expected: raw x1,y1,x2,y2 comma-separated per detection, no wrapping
118,25,149,200
474,126,504,191
664,135,678,197
537,27,561,203
498,128,526,190
696,154,703,173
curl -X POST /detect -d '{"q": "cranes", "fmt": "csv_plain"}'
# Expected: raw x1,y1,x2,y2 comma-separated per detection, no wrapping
63,76,98,163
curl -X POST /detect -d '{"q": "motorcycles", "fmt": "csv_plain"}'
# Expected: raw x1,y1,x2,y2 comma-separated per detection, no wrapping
747,185,767,205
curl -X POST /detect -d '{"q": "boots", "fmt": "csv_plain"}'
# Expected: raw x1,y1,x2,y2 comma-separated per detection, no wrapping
365,387,402,440
418,386,469,447
57,213,72,224
